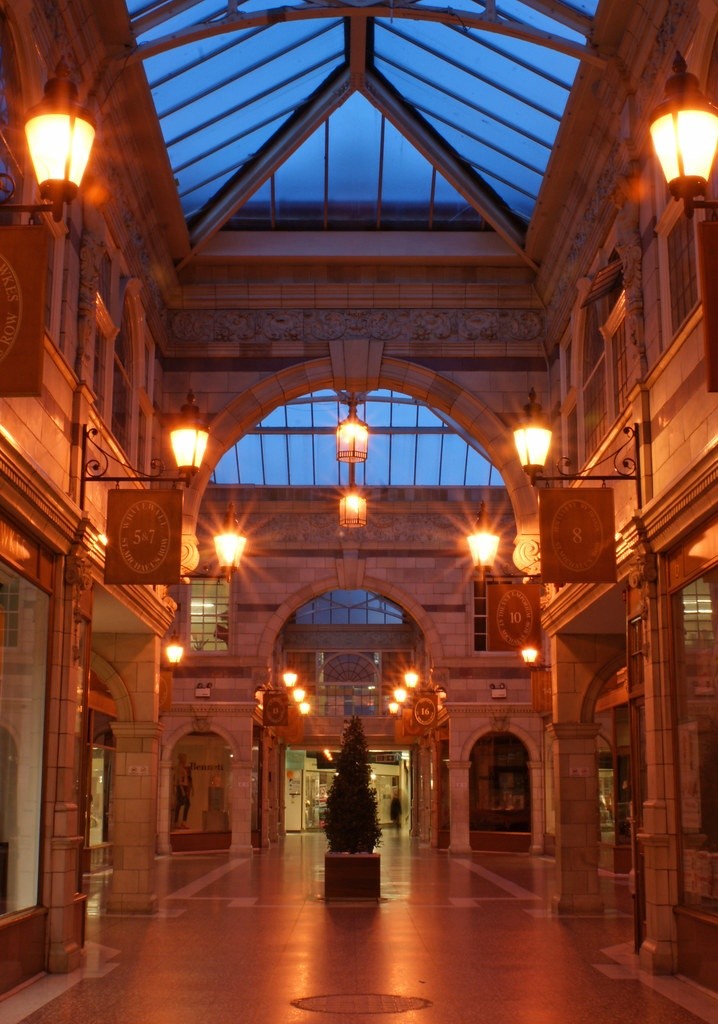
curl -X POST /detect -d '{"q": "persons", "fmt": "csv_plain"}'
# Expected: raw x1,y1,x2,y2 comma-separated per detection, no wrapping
387,792,401,840
175,753,194,829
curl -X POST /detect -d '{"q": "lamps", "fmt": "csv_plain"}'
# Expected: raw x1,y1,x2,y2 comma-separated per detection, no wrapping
385,667,445,718
490,683,507,698
159,629,185,671
512,386,640,487
194,683,212,697
0,54,98,223
333,384,369,463
520,641,551,672
79,390,210,491
466,500,540,580
649,50,718,220
179,501,248,584
339,462,368,528
257,669,310,717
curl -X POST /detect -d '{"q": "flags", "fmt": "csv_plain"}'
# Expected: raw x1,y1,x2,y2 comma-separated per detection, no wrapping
263,694,288,726
0,227,47,398
485,583,543,652
540,488,618,583
411,692,438,728
104,490,182,585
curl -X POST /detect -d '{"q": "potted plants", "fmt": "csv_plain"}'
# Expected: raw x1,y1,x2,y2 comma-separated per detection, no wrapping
324,713,383,901
